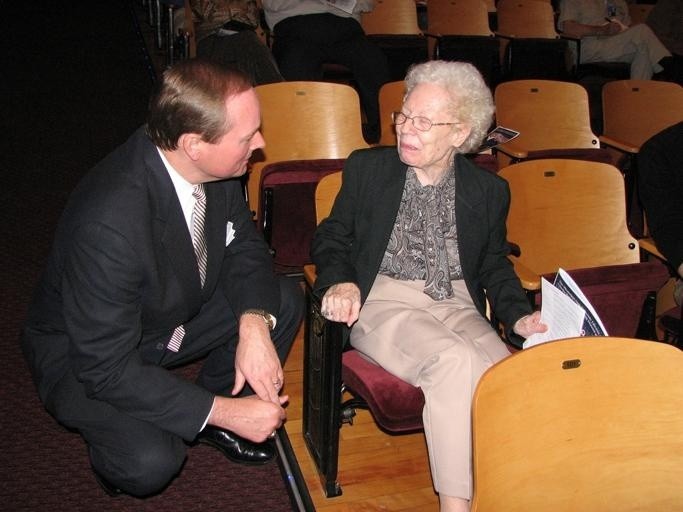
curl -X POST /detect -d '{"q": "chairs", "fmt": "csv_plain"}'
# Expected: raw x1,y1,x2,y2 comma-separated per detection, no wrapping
304,170,513,495
491,159,682,337
234,83,369,286
140,2,682,163
470,337,683,512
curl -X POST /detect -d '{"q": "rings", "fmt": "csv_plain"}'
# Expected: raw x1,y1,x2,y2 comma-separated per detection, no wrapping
274,379,281,387
325,312,333,316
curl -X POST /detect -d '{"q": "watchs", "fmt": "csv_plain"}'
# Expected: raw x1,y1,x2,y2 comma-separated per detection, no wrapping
242,309,272,325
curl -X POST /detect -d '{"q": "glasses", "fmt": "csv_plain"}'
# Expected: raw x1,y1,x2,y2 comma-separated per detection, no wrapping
390,109,462,132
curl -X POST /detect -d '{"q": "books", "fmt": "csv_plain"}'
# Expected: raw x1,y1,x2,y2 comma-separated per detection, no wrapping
553,268,610,336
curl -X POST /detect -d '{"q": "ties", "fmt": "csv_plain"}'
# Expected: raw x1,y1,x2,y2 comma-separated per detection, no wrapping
191,183,209,289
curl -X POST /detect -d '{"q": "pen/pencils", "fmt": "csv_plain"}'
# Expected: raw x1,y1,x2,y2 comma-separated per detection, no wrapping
604,16,612,23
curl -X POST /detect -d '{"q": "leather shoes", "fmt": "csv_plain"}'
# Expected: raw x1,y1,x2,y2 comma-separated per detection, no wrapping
88,444,122,499
194,427,277,466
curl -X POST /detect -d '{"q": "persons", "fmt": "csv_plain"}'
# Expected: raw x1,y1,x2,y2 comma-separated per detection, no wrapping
557,1,673,82
263,0,379,141
636,121,683,307
173,1,287,87
18,55,308,497
310,60,548,512
